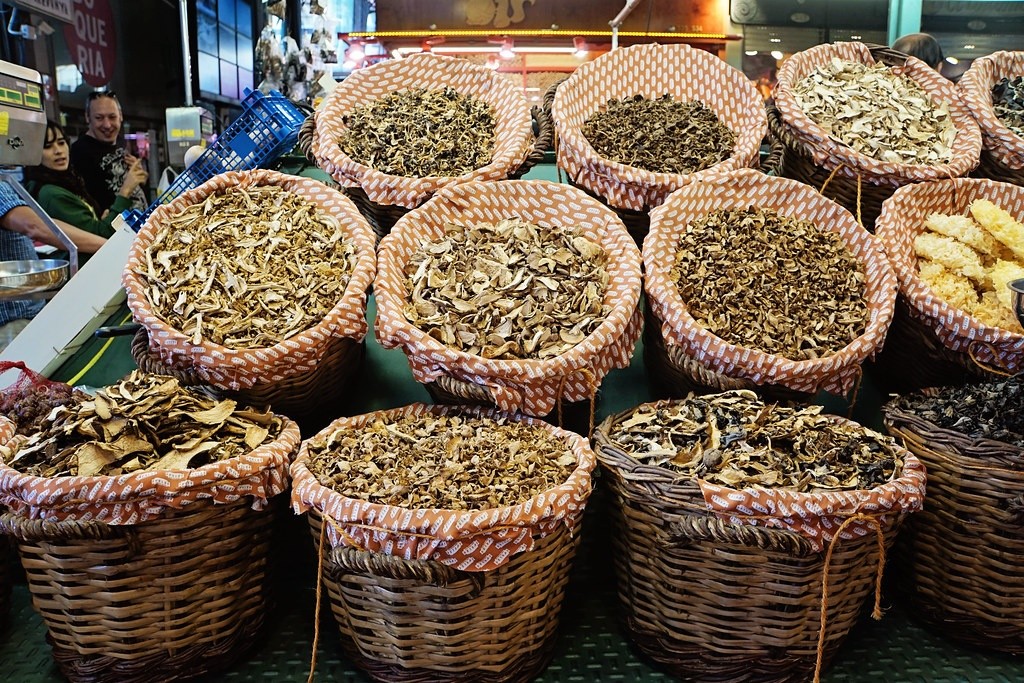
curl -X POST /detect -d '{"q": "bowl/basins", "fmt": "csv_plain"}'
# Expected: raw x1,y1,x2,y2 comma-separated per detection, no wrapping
0,259,69,297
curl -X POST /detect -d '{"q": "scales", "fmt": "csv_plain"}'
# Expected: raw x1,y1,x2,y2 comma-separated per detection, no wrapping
165,106,215,171
0,60,79,302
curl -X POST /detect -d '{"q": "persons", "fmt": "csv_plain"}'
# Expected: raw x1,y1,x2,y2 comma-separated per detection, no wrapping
69,87,151,224
0,165,107,356
892,33,943,73
22,119,148,283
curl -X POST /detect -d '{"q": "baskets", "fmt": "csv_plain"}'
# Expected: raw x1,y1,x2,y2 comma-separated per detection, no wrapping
0,53,1024,683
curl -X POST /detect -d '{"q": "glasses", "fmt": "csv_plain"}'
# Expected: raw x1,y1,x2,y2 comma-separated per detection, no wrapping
88,90,116,99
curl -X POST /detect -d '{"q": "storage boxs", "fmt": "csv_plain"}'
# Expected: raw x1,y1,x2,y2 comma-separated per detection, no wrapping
119,86,311,238
0,225,139,391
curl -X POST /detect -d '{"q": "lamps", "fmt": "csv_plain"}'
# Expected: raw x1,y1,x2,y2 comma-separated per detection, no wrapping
6,4,37,41
